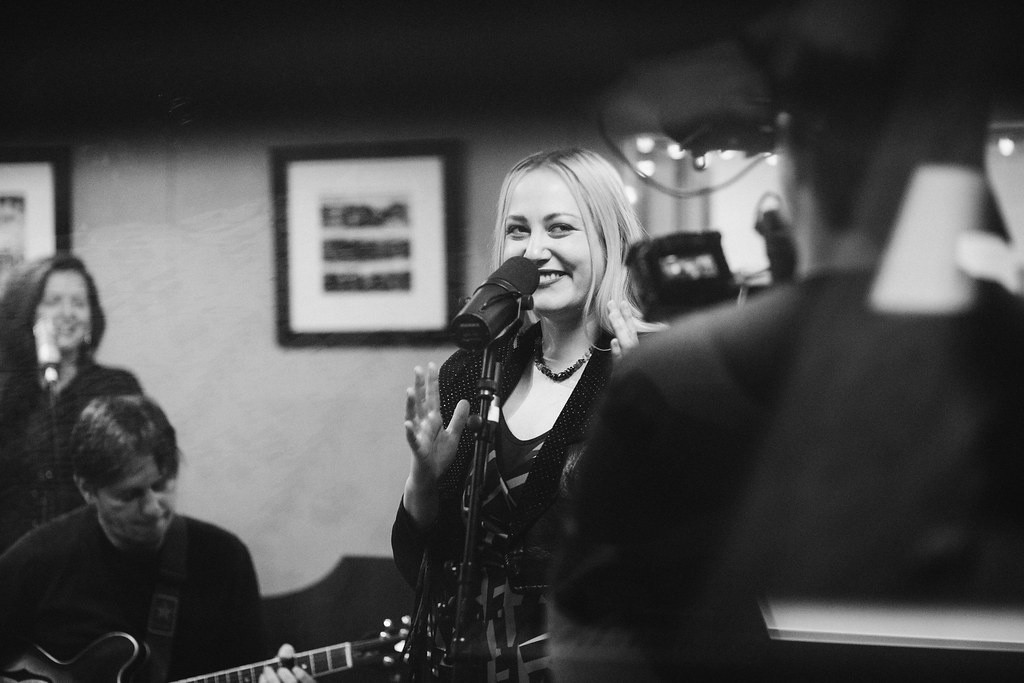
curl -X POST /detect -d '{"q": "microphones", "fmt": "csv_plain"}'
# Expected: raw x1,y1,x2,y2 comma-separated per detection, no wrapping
451,256,541,351
33,319,63,386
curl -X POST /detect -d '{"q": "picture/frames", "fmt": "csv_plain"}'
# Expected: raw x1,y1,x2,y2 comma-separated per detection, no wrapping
0,144,74,299
269,136,464,350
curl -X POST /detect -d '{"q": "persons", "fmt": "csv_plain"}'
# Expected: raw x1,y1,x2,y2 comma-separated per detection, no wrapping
0,392,318,683
0,251,144,555
529,0,1024,683
391,147,662,683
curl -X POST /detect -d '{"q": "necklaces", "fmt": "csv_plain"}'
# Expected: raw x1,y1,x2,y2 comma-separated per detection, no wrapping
533,335,596,383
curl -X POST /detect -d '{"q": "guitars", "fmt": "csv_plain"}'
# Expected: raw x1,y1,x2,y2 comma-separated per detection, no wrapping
0,607,443,683
